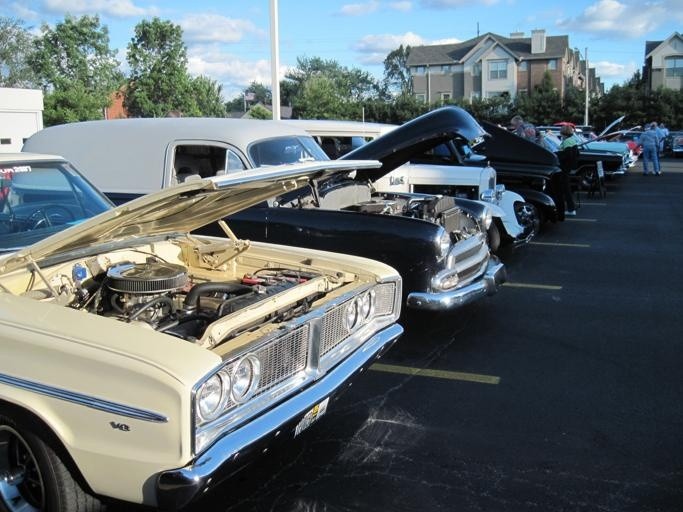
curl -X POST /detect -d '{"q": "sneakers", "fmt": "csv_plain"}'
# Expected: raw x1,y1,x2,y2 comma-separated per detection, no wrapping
564,210,576,216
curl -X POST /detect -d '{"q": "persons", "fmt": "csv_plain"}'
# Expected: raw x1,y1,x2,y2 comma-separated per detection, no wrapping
510,115,598,216
631,122,670,176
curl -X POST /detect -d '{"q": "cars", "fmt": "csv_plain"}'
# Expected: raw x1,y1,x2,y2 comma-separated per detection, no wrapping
2,152,405,508
16,107,508,316
